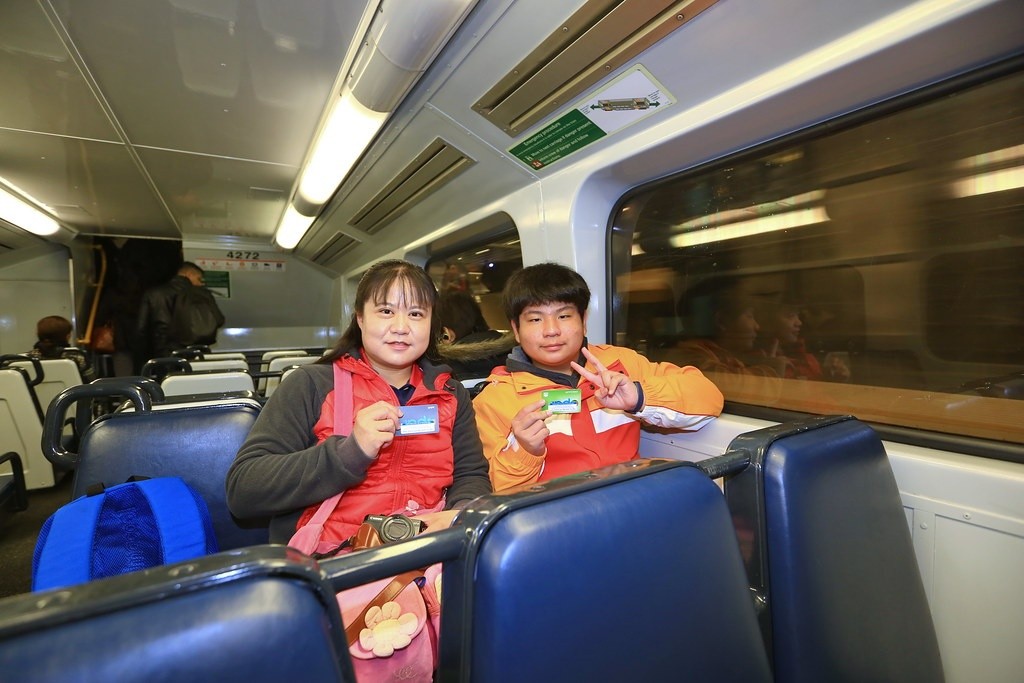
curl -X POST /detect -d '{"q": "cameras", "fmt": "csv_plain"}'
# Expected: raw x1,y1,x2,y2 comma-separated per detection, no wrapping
363,513,428,545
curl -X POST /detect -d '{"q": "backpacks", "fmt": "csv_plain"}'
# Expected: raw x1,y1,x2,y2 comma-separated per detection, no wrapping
31,475,218,595
168,284,224,348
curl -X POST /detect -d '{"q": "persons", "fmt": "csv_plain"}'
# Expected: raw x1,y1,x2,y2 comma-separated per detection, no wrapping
663,290,853,383
433,289,518,382
224,258,493,560
470,263,724,496
32,316,96,385
131,260,224,379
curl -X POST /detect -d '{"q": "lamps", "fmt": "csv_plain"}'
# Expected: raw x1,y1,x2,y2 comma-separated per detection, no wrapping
275,0,474,250
0,177,78,244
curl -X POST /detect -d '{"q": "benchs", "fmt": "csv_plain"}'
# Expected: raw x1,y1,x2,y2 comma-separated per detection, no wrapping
0,347,945,683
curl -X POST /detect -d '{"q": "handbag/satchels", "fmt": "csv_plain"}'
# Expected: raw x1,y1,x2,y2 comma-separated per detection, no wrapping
333,570,436,683
88,322,118,352
